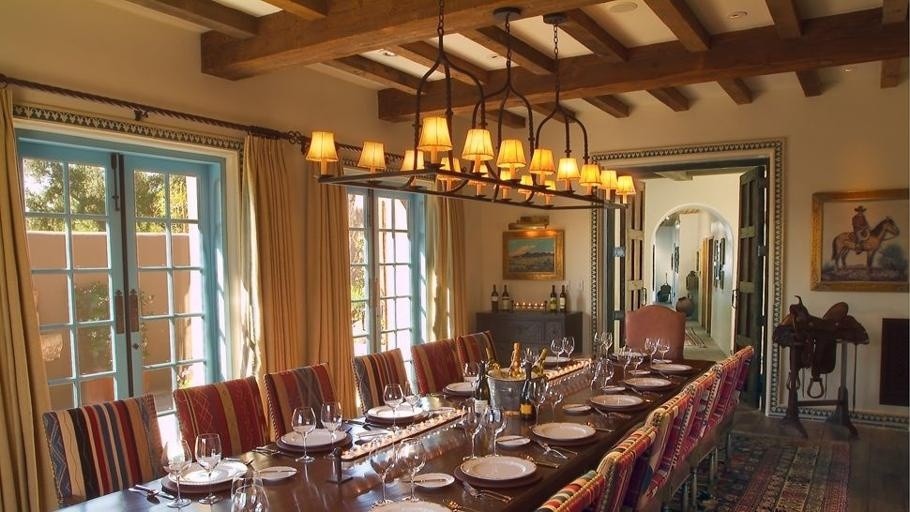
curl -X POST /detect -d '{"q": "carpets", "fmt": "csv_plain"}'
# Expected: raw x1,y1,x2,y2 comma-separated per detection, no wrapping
668,427,851,512
682,322,708,351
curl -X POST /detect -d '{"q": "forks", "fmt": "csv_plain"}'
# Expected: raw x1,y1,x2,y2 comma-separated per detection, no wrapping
462,481,511,503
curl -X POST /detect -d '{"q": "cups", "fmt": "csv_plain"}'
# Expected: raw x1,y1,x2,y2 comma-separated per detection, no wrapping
231,472,264,501
230,486,271,511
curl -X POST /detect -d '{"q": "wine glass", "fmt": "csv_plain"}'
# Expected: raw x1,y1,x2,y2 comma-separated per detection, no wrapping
382,332,671,460
369,433,397,506
320,401,342,460
195,433,223,505
291,407,317,463
162,439,192,508
398,438,425,501
293,463,321,508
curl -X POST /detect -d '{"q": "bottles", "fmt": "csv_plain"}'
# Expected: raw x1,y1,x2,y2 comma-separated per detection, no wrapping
491,284,499,311
330,449,342,478
549,284,557,312
560,284,566,312
502,284,510,312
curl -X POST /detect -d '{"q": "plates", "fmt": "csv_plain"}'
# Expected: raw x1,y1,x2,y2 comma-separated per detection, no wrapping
281,429,347,446
460,455,538,482
368,404,422,420
258,466,297,482
452,466,543,487
276,432,353,453
168,461,247,486
365,411,430,424
372,500,452,512
645,362,694,374
414,473,455,488
160,457,255,492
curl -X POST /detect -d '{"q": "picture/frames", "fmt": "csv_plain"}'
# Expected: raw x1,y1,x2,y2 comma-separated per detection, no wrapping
500,227,567,281
711,237,726,288
810,187,909,293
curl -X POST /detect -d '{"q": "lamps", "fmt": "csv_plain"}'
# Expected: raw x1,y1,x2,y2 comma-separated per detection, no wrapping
304,2,637,211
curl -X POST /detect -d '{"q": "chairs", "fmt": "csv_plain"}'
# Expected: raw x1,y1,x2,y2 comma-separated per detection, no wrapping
535,343,756,509
625,303,688,358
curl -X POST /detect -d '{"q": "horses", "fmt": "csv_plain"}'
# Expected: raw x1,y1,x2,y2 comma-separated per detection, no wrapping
831,215,900,274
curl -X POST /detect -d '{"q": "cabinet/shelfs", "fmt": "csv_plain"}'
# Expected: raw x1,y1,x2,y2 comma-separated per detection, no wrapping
474,306,584,367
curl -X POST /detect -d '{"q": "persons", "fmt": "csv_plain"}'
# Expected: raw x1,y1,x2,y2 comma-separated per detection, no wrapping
850,204,872,255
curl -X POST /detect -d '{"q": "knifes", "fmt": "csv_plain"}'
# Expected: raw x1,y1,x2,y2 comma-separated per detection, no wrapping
258,470,297,473
135,483,175,499
399,478,446,483
256,446,294,457
348,419,383,428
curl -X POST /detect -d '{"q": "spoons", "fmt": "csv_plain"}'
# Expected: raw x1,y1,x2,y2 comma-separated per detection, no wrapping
128,487,160,498
252,449,281,456
443,498,480,511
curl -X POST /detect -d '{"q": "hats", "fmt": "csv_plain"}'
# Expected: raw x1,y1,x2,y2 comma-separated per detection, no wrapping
854,205,867,212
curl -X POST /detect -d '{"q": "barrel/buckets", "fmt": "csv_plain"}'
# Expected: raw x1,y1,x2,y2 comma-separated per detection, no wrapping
485,367,526,412
